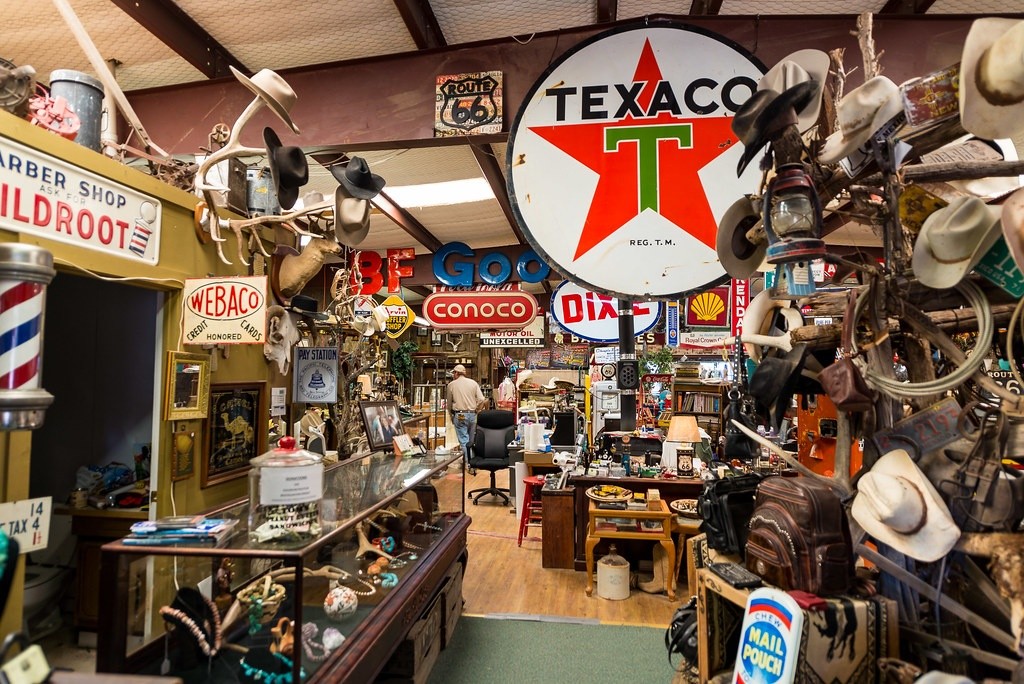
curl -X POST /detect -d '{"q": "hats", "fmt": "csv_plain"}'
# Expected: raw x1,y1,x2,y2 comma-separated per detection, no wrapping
346,316,374,336
368,306,389,332
715,17,1024,432
334,185,370,246
330,156,386,199
229,65,300,135
850,448,961,564
450,365,466,374
262,126,309,210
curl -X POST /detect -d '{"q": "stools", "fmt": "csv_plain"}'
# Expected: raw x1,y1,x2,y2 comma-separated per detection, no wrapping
518,474,546,548
673,517,708,600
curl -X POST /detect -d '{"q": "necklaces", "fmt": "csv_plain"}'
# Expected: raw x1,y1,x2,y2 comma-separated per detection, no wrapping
158,596,223,658
335,574,376,596
240,650,306,684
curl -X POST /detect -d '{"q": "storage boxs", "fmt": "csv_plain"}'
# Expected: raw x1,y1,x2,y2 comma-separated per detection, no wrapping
381,563,466,684
195,152,248,216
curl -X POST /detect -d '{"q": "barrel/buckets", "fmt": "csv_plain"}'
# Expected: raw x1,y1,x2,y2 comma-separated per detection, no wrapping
49,69,106,155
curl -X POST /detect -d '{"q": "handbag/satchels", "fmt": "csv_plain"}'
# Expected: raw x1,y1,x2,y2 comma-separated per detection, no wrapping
927,409,1024,533
697,458,762,556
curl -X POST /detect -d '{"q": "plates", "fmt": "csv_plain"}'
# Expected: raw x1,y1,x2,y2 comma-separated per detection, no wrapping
585,485,632,502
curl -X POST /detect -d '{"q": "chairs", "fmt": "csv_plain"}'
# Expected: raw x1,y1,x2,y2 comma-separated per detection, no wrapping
466,409,514,506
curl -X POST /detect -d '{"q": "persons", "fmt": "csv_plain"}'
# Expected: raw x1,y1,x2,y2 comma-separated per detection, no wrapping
376,414,402,443
447,365,485,475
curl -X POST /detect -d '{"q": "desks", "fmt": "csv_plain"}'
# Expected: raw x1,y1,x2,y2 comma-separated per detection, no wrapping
400,414,432,452
67,506,149,641
570,458,707,573
694,568,899,684
585,488,677,602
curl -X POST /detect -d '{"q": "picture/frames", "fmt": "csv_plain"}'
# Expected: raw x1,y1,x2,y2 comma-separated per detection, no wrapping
201,381,270,489
164,350,212,420
296,347,338,403
416,326,429,337
430,330,443,347
169,429,197,482
359,400,406,453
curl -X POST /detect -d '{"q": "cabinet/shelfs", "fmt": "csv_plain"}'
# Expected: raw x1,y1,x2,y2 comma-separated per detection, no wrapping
540,481,577,571
409,352,446,479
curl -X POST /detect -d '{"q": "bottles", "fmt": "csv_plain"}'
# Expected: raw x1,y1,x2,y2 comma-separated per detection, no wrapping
250,435,324,549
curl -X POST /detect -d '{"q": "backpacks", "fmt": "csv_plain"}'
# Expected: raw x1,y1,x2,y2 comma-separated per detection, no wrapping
743,475,857,596
665,599,699,672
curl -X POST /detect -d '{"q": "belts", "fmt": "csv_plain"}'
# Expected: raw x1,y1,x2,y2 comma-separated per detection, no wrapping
456,410,476,413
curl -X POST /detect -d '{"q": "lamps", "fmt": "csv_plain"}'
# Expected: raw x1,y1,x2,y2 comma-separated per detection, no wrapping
667,415,704,478
760,162,828,263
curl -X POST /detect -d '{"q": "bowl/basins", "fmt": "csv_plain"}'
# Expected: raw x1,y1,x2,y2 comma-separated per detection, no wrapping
670,498,702,519
